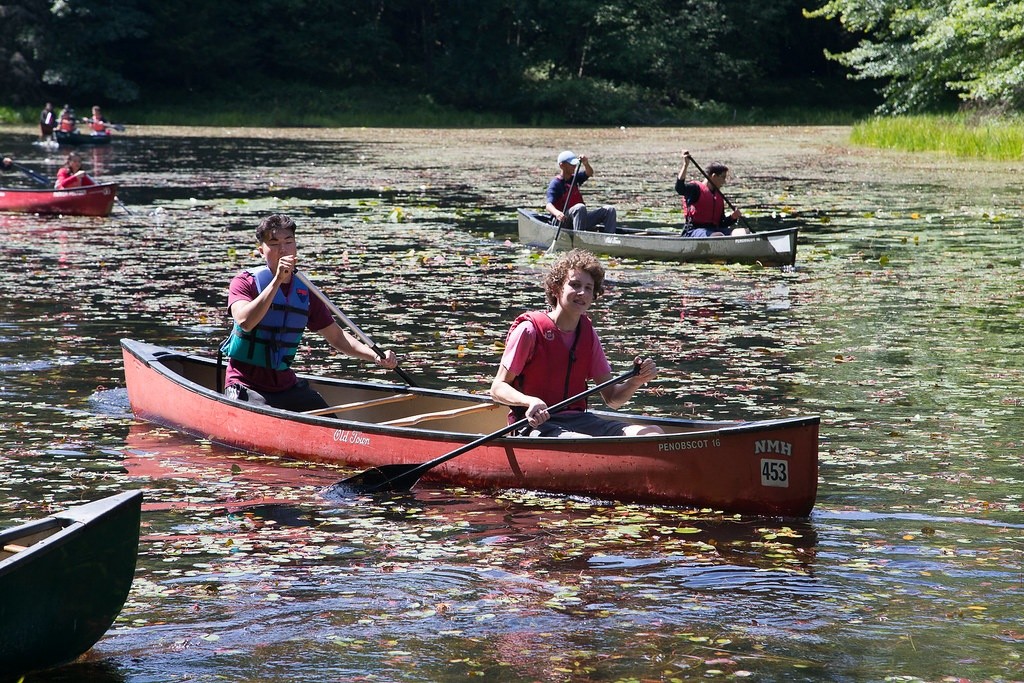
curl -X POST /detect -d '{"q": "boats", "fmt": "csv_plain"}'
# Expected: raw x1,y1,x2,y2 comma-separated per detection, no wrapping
0,489,143,680
40,121,110,142
0,181,126,217
121,336,821,516
517,206,797,267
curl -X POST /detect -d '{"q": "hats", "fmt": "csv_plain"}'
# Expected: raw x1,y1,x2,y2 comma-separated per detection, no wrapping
558,151,579,165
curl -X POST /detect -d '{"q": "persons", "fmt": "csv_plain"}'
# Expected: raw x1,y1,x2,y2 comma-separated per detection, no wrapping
490,248,665,437
0,153,12,170
224,214,398,418
675,150,750,236
54,151,95,190
546,151,617,233
42,102,80,135
83,106,111,135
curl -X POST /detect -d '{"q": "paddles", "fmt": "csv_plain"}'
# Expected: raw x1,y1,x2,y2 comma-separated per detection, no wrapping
544,156,582,255
11,162,51,188
331,359,646,497
685,152,756,233
84,173,133,216
290,268,420,387
88,119,126,132
